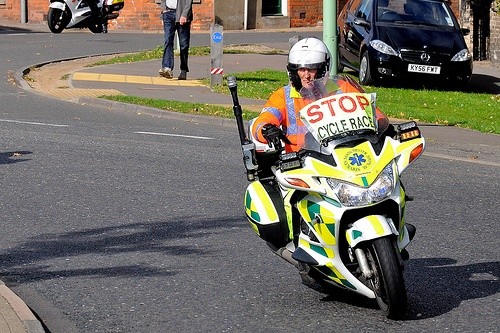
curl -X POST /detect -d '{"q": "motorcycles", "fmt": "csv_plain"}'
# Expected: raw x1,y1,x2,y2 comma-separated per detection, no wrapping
227,74,426,319
47,0,124,33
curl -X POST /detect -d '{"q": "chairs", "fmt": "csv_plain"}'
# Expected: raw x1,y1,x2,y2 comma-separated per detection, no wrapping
404,4,420,14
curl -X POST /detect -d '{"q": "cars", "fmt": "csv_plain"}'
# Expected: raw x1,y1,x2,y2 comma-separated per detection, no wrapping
337,0,473,91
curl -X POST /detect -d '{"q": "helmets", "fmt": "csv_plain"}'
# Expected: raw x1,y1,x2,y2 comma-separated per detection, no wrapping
287,38,332,87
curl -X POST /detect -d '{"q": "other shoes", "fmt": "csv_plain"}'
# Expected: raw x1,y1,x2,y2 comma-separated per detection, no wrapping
102,23,107,33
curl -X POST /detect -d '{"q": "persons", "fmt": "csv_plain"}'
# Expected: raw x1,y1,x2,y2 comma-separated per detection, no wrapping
159,0,193,80
252,37,390,251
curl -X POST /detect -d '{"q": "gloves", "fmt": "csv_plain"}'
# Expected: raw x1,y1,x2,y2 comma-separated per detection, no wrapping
262,123,292,150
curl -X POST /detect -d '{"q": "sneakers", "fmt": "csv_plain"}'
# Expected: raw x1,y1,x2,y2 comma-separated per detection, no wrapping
178,73,186,80
159,68,173,78
